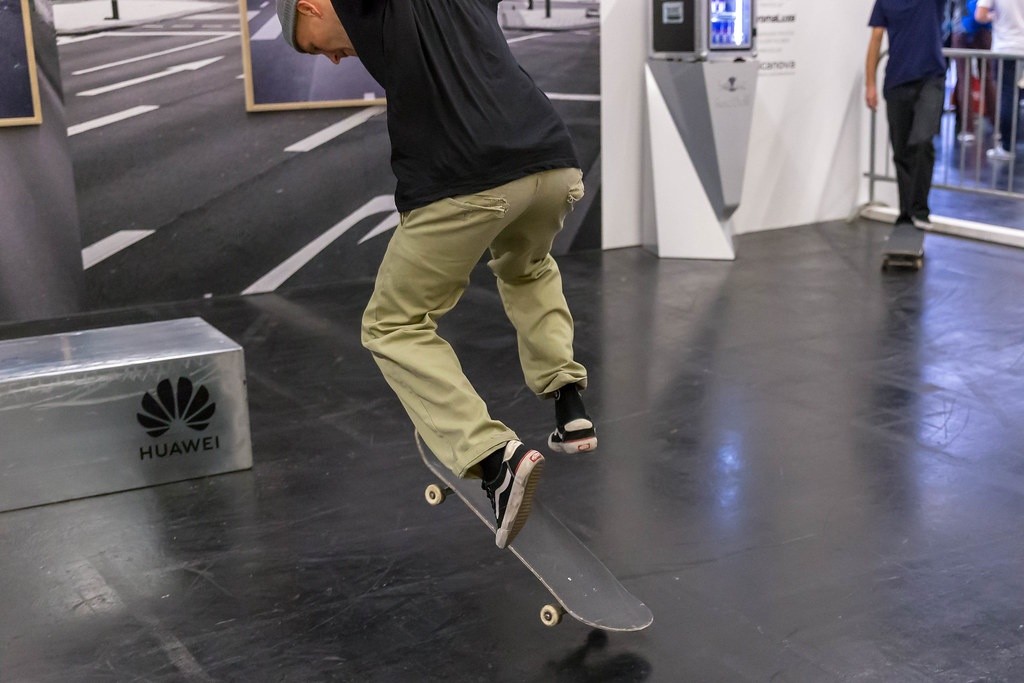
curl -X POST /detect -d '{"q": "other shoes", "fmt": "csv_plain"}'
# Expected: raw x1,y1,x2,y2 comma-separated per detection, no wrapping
911,214,933,231
957,131,975,142
987,147,1011,160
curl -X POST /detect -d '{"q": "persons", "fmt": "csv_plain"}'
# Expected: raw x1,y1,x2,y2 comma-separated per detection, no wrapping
936,0,1024,160
276,0,598,550
865,0,947,231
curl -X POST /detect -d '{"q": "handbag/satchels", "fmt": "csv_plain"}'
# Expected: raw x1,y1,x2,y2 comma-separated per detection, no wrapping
960,29,991,50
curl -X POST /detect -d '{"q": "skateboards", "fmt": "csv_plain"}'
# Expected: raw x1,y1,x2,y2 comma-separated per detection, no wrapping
879,220,927,271
406,422,655,635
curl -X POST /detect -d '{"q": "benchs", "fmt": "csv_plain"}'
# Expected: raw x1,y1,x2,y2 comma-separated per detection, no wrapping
0,317,252,514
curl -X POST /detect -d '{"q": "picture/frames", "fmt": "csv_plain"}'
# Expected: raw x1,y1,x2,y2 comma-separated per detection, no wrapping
237,0,387,114
0,0,44,128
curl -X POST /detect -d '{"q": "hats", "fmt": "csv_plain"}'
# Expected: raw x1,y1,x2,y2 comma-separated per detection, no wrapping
275,0,304,54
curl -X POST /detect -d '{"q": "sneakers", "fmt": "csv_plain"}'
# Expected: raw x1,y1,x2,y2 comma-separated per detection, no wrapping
548,409,598,454
481,439,545,549
